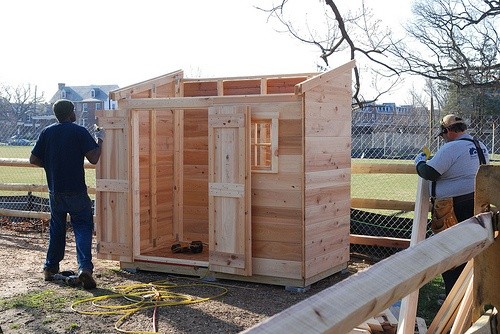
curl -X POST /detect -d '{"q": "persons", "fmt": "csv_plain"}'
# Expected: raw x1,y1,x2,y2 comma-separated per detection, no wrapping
414,113,489,305
29,99,105,290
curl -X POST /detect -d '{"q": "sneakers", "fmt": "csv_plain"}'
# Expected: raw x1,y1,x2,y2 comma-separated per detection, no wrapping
78,268,96,290
44,268,58,281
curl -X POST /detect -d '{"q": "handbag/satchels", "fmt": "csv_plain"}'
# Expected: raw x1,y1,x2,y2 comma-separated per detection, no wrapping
431,198,458,234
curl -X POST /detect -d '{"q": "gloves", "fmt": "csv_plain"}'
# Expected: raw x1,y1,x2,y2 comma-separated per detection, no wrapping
94,126,104,141
414,152,426,166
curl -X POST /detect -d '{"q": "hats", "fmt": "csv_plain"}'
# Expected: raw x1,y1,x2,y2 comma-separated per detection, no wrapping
53,100,74,123
434,115,465,140
